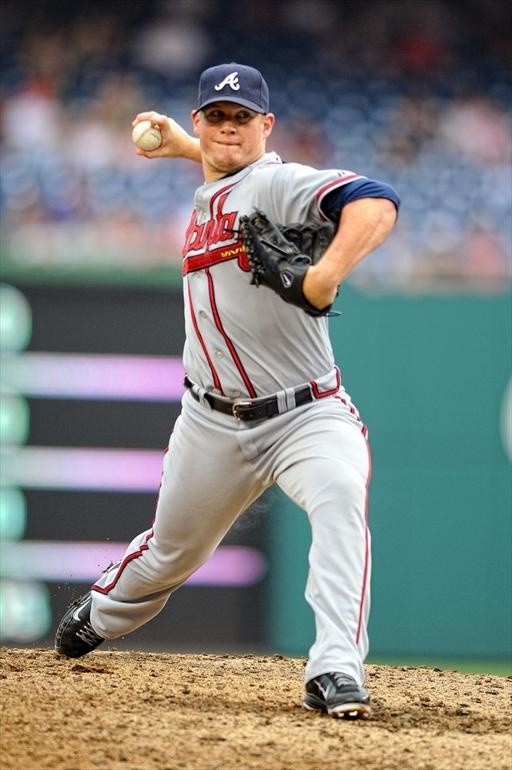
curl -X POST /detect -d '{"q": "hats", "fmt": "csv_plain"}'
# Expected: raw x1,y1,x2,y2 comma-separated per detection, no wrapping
193,63,271,116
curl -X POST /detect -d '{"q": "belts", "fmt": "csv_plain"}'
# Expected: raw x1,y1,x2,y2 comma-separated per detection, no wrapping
181,377,318,422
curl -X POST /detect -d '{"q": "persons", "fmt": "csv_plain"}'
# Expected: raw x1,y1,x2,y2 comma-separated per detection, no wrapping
53,62,400,722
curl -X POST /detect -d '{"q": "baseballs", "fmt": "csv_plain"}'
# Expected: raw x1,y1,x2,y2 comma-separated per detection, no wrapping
132,119,161,153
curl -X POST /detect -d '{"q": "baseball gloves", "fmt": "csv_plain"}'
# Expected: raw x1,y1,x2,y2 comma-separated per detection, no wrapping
239,212,335,317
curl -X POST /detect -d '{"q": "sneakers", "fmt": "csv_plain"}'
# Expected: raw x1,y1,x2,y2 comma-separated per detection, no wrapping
54,588,106,659
301,667,373,720
